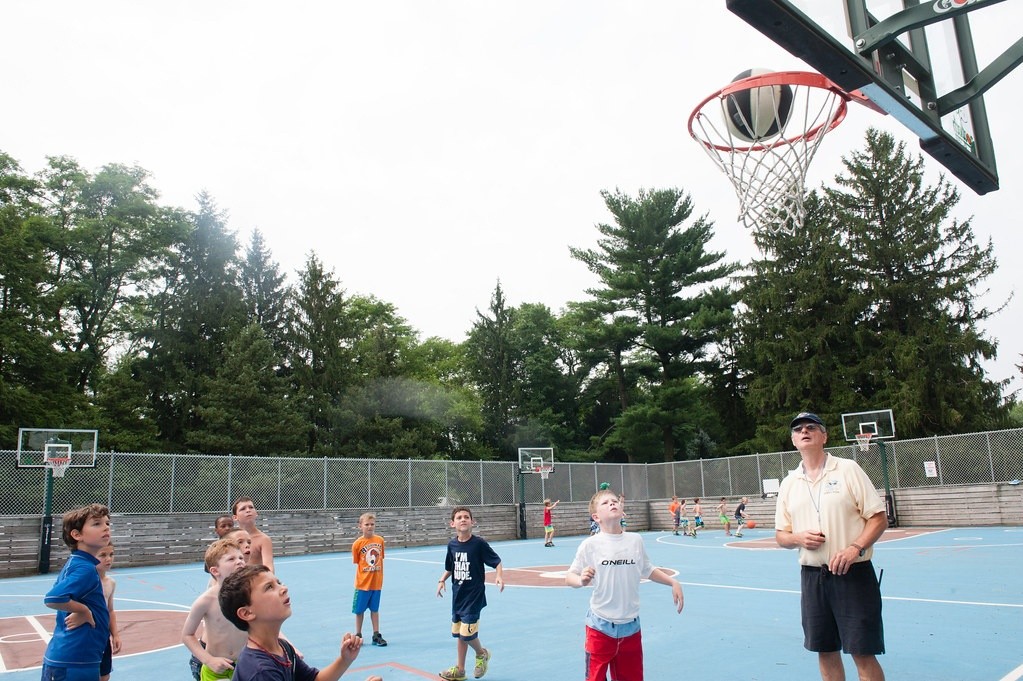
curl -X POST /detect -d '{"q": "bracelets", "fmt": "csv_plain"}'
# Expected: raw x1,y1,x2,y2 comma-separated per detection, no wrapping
439,580,445,582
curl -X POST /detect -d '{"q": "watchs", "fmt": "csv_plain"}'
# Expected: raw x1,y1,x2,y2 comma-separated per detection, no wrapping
850,543,866,557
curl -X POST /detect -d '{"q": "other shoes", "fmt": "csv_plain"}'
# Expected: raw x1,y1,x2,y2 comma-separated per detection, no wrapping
691,533,696,538
692,528,697,535
734,533,742,537
545,542,554,547
726,532,732,536
673,530,681,535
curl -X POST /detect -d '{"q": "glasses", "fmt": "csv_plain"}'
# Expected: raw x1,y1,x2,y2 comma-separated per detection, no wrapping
793,423,823,431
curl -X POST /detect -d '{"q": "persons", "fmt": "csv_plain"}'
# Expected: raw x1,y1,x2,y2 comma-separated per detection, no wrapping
565,490,684,681
774,412,889,681
352,513,388,646
181,497,273,681
41,503,112,681
669,496,751,539
219,564,383,681
589,482,628,537
435,507,505,681
95,541,122,681
543,498,560,547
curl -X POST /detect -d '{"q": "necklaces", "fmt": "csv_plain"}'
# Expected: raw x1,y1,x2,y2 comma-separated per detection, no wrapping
248,636,292,668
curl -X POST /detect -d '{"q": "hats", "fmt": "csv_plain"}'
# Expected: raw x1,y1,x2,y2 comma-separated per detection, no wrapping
600,482,611,489
790,412,824,428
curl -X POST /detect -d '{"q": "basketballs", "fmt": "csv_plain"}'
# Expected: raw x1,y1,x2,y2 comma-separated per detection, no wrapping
747,520,755,529
724,68,797,142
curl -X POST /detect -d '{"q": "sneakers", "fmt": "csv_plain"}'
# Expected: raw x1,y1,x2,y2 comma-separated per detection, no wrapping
474,648,492,678
439,666,467,680
356,633,362,638
372,632,387,646
